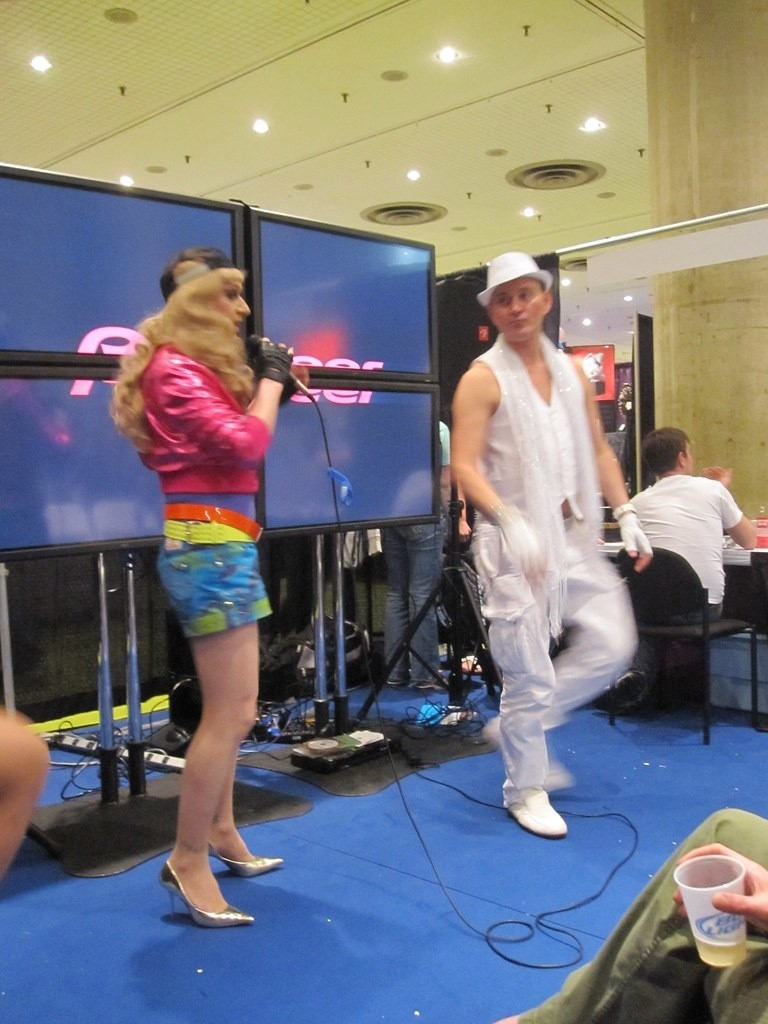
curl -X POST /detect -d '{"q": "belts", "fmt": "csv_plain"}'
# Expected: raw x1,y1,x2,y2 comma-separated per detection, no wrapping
162,502,263,544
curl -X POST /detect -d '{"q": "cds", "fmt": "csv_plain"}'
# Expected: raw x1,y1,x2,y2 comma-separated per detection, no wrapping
308,739,338,749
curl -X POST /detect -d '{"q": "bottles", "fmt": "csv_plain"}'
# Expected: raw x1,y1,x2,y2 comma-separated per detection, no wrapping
753,507,768,549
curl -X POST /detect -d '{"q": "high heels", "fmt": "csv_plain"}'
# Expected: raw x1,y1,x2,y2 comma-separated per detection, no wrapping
160,861,255,927
210,844,283,876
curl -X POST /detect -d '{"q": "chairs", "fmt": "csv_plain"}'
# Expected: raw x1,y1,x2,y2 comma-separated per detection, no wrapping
608,548,757,746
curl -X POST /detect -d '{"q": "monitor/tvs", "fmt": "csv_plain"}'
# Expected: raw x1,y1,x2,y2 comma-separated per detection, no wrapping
0,164,441,562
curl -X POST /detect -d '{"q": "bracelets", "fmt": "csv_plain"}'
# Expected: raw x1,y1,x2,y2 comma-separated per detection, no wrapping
613,504,638,520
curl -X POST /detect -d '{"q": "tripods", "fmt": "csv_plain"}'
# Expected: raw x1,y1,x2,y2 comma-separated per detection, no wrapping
346,470,503,733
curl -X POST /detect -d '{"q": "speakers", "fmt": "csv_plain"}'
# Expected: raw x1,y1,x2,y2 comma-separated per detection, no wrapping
165,583,281,730
435,273,499,426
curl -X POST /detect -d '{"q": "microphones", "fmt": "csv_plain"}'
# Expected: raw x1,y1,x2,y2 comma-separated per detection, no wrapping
246,334,314,402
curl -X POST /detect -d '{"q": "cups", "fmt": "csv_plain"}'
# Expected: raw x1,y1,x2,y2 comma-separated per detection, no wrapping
674,854,747,968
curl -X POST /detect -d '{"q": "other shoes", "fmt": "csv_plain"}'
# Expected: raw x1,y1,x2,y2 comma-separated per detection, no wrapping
508,790,567,836
484,717,575,790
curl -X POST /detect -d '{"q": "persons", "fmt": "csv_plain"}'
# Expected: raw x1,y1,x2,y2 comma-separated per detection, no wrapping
452,252,656,841
0,706,51,885
113,248,310,929
629,426,757,621
382,419,451,692
494,807,768,1023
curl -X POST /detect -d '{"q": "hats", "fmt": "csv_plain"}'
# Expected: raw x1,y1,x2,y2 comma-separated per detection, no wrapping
477,252,553,306
161,248,234,302
